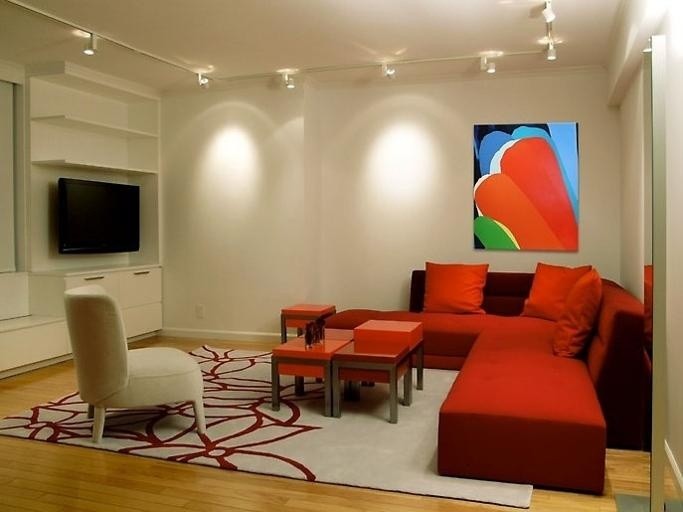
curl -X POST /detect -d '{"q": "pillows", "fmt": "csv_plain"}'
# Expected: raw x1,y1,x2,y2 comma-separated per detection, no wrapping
520,260,593,320
551,267,602,357
424,260,489,316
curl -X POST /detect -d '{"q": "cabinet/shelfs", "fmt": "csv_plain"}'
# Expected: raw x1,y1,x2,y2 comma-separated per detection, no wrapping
120,263,162,340
31,112,161,177
29,268,120,358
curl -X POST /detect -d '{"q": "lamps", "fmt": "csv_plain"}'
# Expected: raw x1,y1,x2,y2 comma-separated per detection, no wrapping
13,0,557,90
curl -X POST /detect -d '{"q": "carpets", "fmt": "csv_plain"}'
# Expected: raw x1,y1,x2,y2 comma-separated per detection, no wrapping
0,343,535,508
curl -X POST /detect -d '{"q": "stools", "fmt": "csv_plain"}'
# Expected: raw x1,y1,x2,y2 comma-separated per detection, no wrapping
271,302,423,424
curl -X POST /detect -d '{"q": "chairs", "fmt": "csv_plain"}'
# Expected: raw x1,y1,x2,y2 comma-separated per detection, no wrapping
61,284,205,444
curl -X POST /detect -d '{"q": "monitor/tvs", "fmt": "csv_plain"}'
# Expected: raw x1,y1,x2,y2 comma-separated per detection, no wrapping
58,177,140,254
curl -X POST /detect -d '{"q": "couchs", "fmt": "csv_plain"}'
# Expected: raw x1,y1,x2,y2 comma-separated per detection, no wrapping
325,270,644,498
